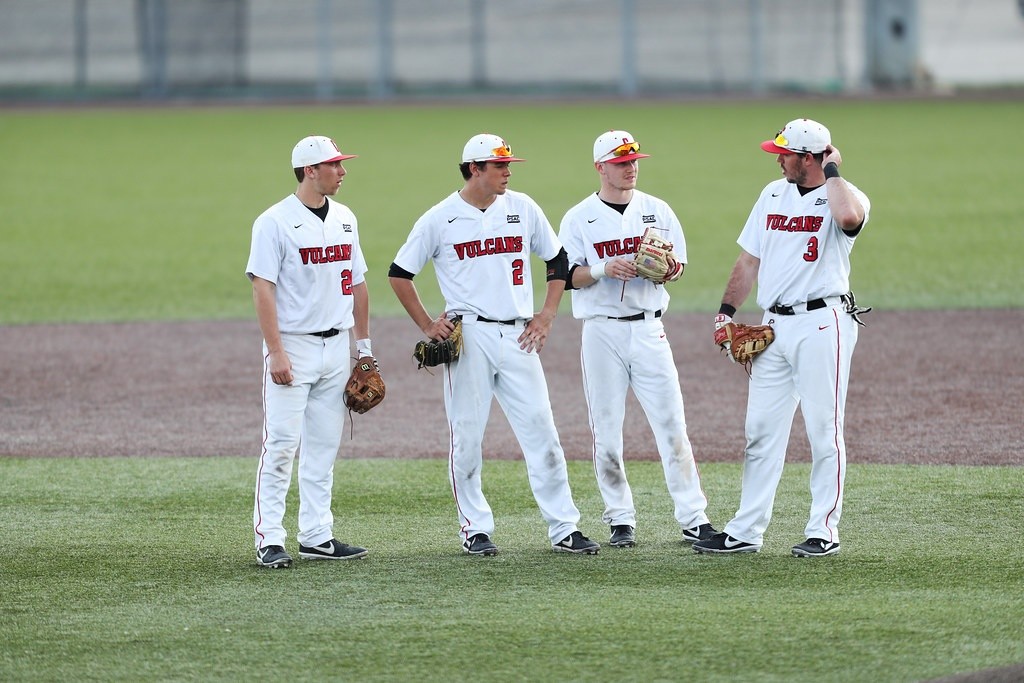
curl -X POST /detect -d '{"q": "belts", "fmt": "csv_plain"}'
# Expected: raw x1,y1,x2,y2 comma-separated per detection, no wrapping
607,308,662,322
309,328,340,338
453,315,526,325
769,295,844,315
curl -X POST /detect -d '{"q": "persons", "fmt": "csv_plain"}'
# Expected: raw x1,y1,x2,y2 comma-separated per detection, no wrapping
245,136,385,568
558,130,722,547
692,119,870,557
387,133,601,556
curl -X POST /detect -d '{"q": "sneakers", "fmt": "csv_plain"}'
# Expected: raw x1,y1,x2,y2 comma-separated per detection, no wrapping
463,534,499,557
682,524,722,543
256,545,292,570
298,539,367,560
791,538,840,558
551,532,601,555
692,532,762,555
609,525,637,548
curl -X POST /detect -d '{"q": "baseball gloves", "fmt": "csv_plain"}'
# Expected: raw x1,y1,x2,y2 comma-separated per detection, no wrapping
414,317,462,366
630,227,676,285
345,356,385,414
713,321,774,363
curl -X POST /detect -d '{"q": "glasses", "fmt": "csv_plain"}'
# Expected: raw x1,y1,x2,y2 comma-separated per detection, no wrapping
471,145,513,158
774,133,812,153
597,142,640,162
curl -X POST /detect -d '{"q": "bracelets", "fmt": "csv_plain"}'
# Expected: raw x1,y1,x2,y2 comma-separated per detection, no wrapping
718,303,737,318
823,162,840,180
590,262,607,280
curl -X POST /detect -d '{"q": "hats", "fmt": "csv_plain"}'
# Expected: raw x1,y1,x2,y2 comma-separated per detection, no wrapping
761,118,830,155
291,135,356,169
592,130,650,164
462,134,527,162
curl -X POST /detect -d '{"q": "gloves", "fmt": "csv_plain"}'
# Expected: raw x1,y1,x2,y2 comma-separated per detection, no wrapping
843,290,872,328
